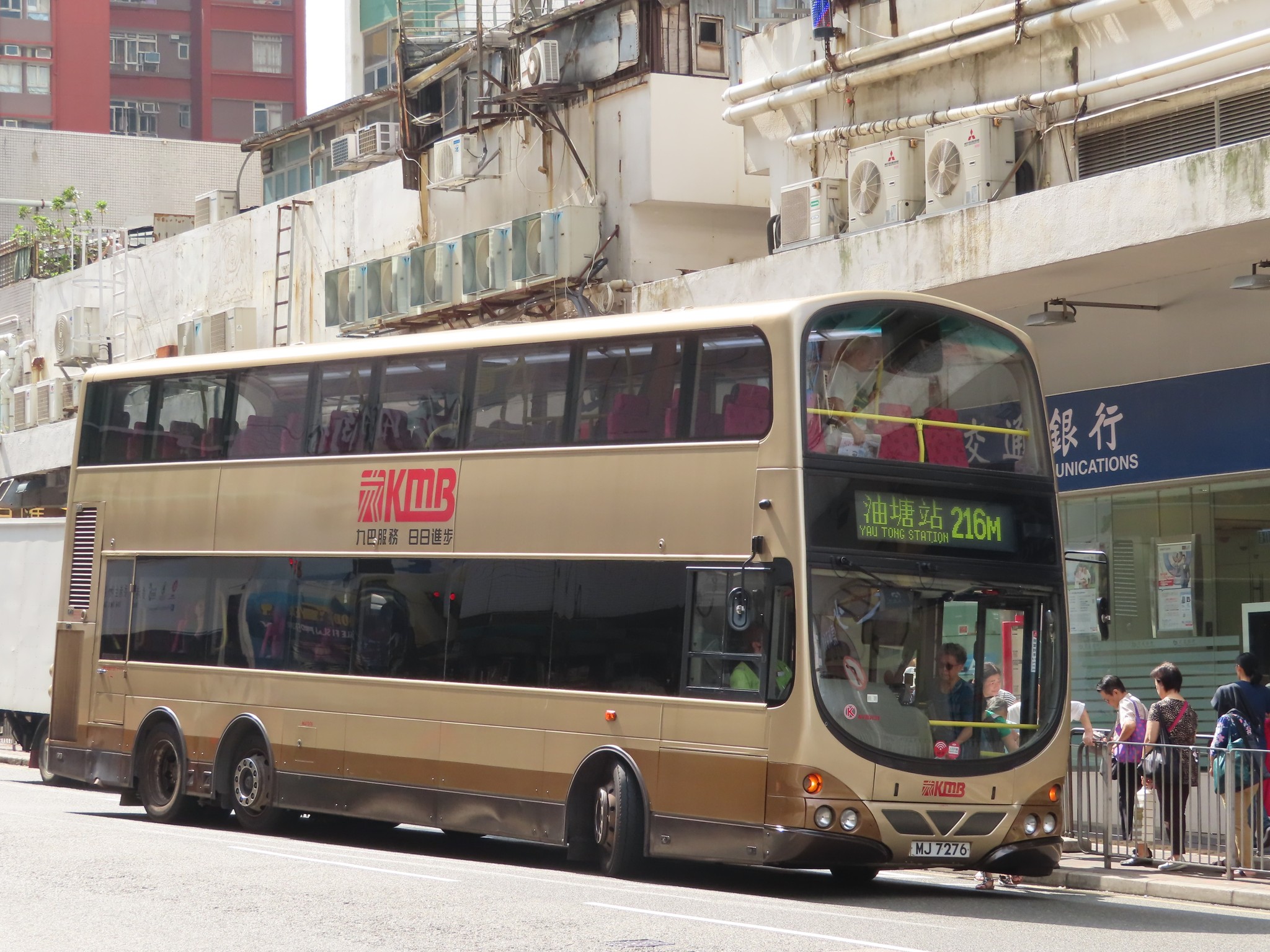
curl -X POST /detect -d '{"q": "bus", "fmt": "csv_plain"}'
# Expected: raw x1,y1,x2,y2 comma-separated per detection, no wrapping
48,291,1111,878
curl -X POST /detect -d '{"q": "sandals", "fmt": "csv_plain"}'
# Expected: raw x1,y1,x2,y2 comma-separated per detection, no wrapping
975,877,995,890
998,874,1017,887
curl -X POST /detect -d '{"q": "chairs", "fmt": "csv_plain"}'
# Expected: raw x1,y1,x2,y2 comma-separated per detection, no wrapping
120,384,969,467
176,608,339,662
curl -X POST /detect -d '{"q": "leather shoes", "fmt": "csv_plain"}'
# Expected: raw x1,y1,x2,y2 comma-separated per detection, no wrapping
1120,857,1154,867
1130,848,1153,858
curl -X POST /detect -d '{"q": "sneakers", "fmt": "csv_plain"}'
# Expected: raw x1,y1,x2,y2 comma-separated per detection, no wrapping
1158,857,1188,871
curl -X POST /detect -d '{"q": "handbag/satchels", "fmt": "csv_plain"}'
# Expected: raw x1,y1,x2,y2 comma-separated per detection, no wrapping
1137,704,1185,785
1214,714,1270,795
1100,751,1118,781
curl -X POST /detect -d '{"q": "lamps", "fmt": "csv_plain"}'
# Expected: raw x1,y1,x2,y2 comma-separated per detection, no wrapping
1229,260,1270,290
1025,298,1160,327
16,480,41,493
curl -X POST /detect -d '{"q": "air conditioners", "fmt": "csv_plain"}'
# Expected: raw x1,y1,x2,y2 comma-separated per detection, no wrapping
331,133,365,170
781,177,848,247
925,114,1014,215
338,206,603,332
145,52,161,64
434,133,478,186
176,305,257,357
519,39,559,88
848,136,925,231
10,305,100,430
4,119,18,128
142,103,155,113
3,45,52,58
194,190,240,228
357,120,400,161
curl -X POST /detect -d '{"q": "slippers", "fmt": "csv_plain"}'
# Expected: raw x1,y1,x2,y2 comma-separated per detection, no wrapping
974,871,984,882
1009,875,1023,885
1221,869,1254,879
1213,860,1225,867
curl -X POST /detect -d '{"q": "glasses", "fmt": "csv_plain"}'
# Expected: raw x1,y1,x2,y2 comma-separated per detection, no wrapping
1105,694,1111,703
936,662,959,671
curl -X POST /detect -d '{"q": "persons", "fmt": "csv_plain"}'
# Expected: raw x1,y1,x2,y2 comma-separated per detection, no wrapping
821,336,883,454
1176,554,1190,588
1211,652,1270,851
176,597,206,651
928,643,975,760
1096,675,1152,867
730,629,792,699
967,662,1094,890
1210,683,1267,877
1074,564,1091,589
1141,661,1198,871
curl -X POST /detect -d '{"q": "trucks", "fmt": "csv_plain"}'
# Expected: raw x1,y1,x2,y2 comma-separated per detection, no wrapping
1,518,81,786
